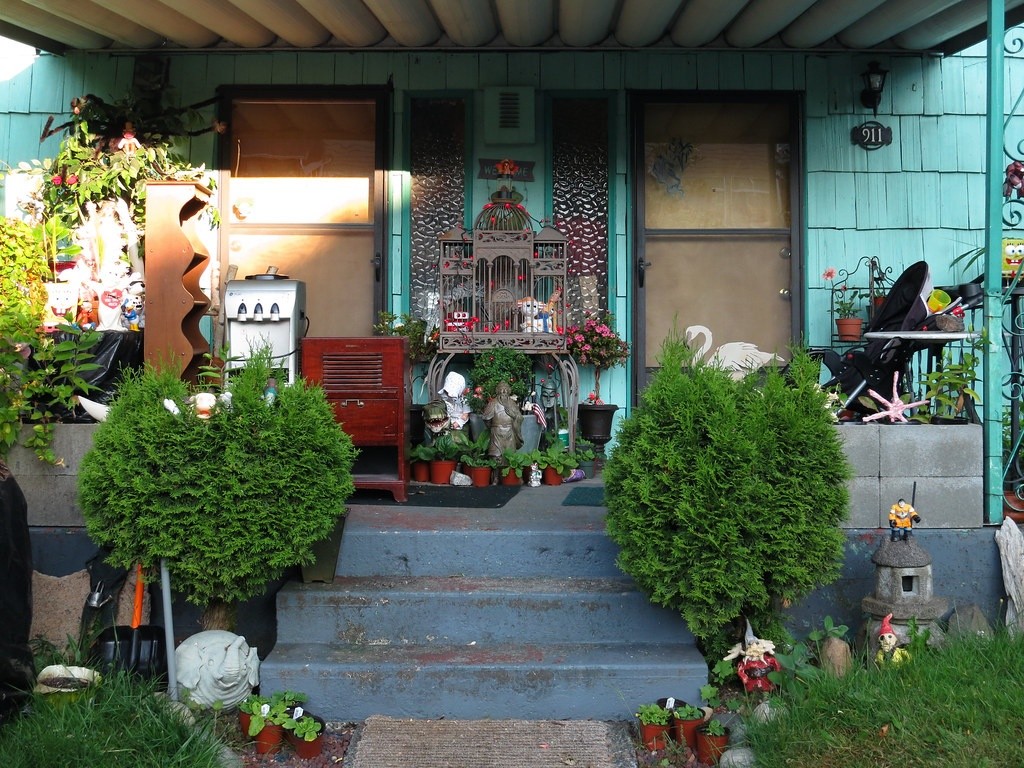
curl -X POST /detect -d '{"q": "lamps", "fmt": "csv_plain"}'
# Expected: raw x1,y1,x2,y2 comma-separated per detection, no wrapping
857,57,891,122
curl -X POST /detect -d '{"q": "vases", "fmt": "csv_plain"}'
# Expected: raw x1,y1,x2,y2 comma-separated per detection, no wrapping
832,318,865,343
468,411,544,454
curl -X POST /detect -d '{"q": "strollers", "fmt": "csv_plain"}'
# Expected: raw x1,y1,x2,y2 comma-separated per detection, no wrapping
774,259,985,424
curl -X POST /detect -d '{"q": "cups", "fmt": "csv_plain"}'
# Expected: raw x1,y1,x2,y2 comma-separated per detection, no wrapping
928,289,951,314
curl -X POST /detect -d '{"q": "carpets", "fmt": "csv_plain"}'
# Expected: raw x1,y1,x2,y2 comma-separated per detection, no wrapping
562,483,612,509
344,485,523,511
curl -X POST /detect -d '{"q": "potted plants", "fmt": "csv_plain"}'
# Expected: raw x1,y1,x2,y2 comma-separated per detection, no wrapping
523,449,544,484
538,443,580,486
499,448,525,486
454,429,490,479
635,703,672,752
248,702,285,755
236,694,273,741
695,718,731,766
282,714,328,759
410,442,434,482
460,453,498,488
576,403,620,455
671,703,706,748
929,324,993,427
1001,391,1024,526
429,434,457,485
858,278,889,307
575,436,596,480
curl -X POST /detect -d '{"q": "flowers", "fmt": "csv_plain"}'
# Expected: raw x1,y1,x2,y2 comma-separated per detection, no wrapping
566,314,633,404
820,266,862,318
464,347,538,414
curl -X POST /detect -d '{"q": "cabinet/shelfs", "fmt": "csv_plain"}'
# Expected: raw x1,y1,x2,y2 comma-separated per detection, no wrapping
142,175,215,391
299,331,413,507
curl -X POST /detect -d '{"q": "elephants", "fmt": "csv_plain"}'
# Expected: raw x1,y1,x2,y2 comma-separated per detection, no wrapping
173,625,260,715
